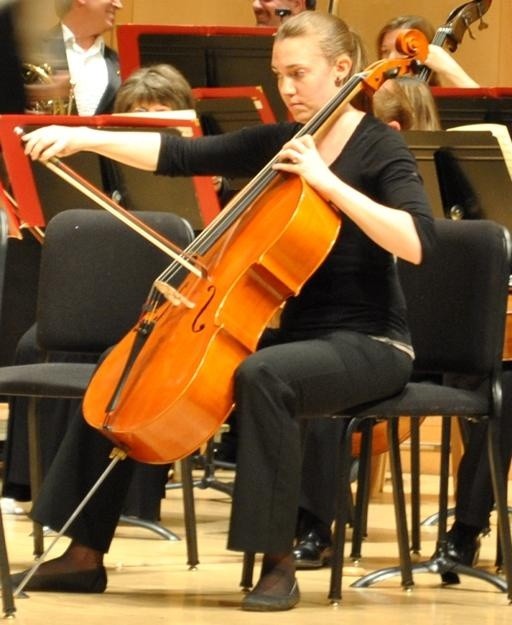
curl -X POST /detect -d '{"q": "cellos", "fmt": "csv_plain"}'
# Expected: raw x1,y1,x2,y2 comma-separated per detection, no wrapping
349,0,491,458
82,30,429,464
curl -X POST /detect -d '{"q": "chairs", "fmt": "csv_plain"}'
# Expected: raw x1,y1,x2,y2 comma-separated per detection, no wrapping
241,220,510,606
1,208,201,568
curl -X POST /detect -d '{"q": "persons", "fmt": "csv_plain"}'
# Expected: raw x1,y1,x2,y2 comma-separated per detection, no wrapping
39,0,122,117
378,14,482,88
252,0,317,28
22,7,437,611
284,73,511,574
0,65,244,518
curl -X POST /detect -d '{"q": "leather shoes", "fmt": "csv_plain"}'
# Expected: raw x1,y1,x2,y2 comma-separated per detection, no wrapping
431,530,481,565
291,525,333,568
9,564,106,593
241,576,301,612
